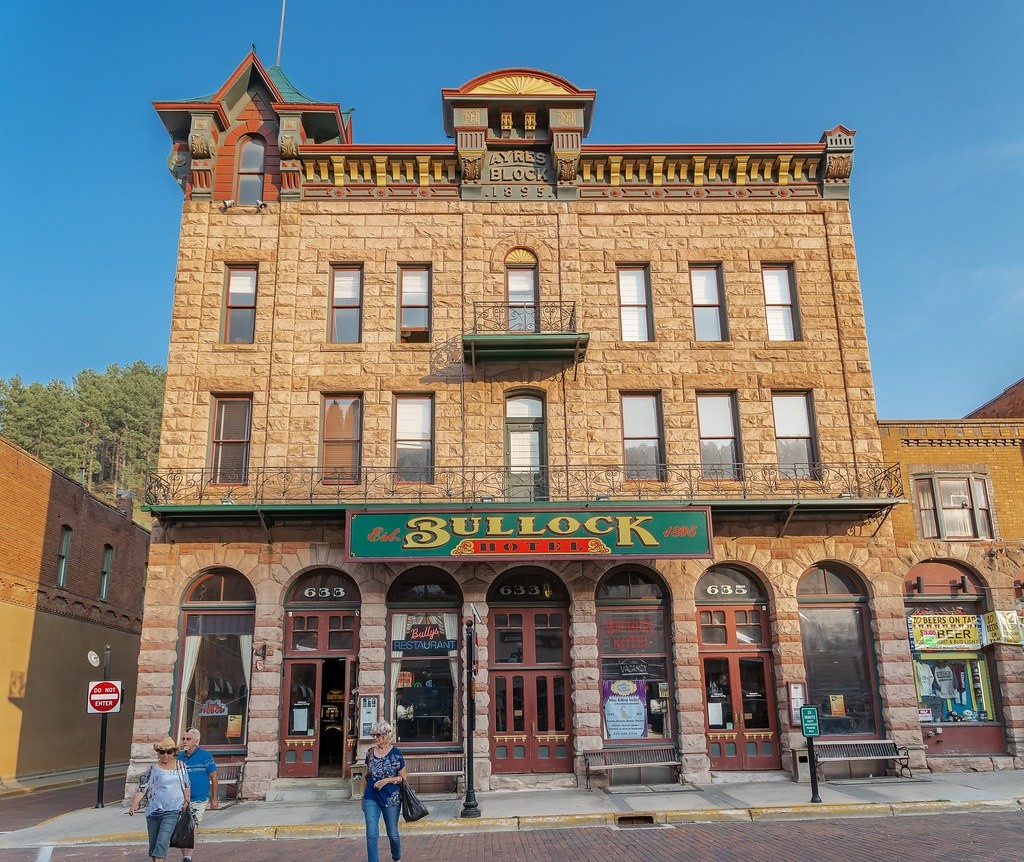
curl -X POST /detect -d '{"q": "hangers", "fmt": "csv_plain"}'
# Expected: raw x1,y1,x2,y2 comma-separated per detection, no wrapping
935,660,945,664
917,661,924,664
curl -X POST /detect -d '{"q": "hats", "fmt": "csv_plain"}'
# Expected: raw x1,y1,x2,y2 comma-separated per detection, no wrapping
153,737,180,751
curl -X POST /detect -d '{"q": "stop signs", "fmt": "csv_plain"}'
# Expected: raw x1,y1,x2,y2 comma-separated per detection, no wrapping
86,681,122,714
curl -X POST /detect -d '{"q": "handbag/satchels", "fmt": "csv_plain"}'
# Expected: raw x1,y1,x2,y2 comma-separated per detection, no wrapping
169,801,194,849
396,775,429,823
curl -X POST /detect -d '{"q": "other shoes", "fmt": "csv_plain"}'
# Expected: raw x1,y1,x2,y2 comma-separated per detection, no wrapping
182,857,192,862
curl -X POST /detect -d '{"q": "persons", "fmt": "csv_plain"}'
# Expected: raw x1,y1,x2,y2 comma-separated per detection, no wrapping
128,737,191,862
174,727,218,862
361,720,407,862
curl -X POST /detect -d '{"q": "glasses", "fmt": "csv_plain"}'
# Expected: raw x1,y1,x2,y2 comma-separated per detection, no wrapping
370,733,381,738
159,750,173,754
182,737,196,741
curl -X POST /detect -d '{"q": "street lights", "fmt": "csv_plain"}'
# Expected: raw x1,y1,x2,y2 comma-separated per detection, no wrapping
87,643,112,809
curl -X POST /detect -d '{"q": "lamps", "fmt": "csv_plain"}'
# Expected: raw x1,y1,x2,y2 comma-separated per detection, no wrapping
510,642,524,660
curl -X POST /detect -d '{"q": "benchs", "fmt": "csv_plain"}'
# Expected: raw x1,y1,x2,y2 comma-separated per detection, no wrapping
584,745,684,792
402,754,468,795
137,761,244,806
805,740,913,782
